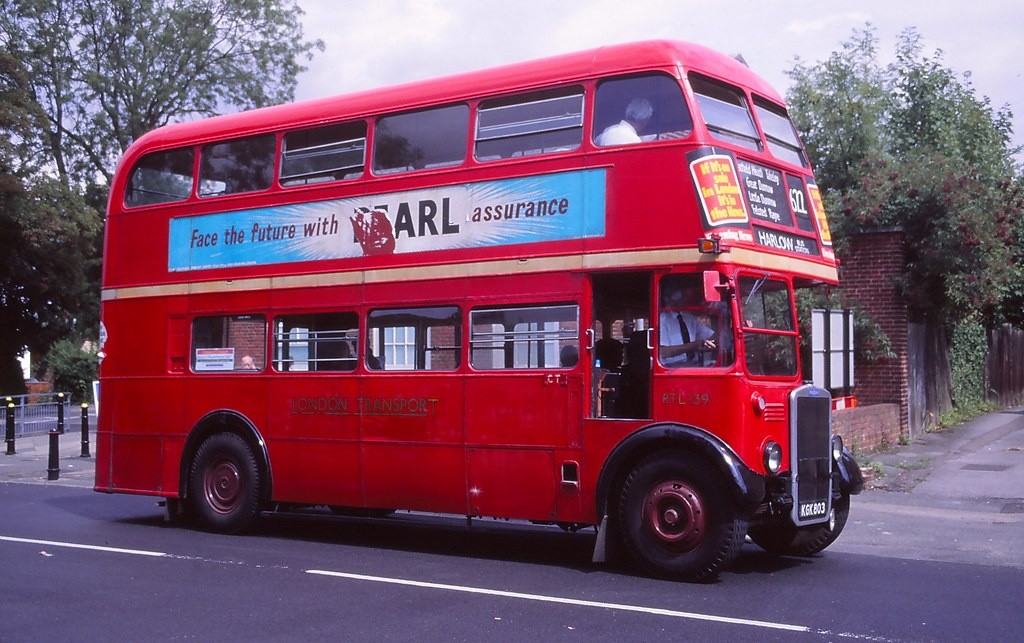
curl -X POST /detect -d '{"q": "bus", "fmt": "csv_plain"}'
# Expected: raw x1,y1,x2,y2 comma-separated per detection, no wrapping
89,34,864,580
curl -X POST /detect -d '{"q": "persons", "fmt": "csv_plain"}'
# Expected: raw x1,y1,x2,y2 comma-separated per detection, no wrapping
658,288,716,367
561,345,578,367
596,339,624,369
593,97,653,147
242,356,256,370
334,335,382,370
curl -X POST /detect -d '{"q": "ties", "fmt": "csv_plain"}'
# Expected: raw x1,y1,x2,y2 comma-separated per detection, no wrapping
677,314,695,361
